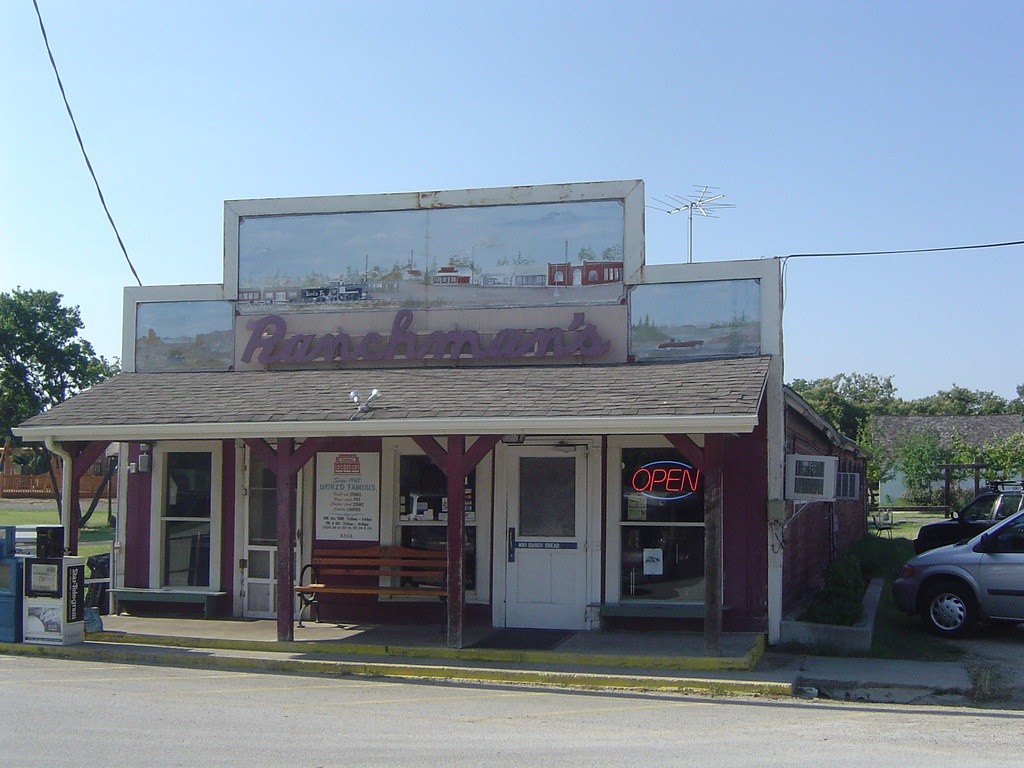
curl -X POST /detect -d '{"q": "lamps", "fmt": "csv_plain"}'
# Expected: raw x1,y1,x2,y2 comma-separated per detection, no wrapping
349,388,381,412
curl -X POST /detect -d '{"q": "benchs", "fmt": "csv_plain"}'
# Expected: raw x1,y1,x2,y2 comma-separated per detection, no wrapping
106,587,228,620
293,545,448,635
587,600,731,636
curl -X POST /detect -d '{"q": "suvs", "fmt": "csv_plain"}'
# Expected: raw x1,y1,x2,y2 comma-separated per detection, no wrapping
913,478,1024,555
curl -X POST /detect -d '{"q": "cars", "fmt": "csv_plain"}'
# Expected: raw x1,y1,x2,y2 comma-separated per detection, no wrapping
892,507,1024,636
620,457,703,550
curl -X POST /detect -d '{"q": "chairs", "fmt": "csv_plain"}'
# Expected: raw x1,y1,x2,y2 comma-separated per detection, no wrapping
872,514,892,540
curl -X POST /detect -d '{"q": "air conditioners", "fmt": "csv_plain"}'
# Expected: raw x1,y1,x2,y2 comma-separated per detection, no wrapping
784,454,860,502
